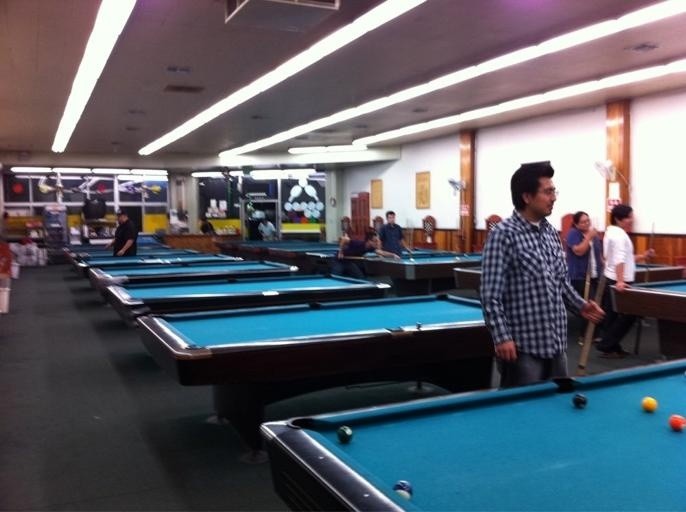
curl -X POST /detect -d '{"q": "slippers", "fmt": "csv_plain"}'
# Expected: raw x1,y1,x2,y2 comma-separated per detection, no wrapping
597,351,623,359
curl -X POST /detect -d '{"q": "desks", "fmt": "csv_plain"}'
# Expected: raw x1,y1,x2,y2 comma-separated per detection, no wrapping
102,273,390,323
64,232,483,292
136,289,495,454
609,277,686,361
257,358,685,512
636,262,686,283
63,231,582,512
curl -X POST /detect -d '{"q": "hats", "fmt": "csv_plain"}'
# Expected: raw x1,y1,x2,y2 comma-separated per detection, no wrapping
117,208,127,215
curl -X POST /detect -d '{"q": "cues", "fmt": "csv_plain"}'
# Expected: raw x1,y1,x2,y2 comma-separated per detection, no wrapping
634,223,655,354
583,245,593,302
577,273,607,370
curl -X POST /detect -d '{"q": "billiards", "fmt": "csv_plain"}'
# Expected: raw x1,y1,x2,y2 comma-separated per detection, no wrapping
669,414,686,431
337,426,353,442
573,393,588,408
393,480,412,502
456,257,460,260
409,257,414,262
643,397,656,412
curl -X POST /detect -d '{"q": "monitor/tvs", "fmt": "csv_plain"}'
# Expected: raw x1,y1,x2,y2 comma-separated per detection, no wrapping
82,199,106,219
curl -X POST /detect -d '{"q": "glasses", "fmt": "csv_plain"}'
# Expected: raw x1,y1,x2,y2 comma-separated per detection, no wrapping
537,188,559,195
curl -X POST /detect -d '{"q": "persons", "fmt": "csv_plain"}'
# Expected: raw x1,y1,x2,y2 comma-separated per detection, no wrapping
479,161,605,387
378,212,413,292
567,213,607,349
101,209,138,257
591,205,657,356
258,214,277,242
337,227,401,279
